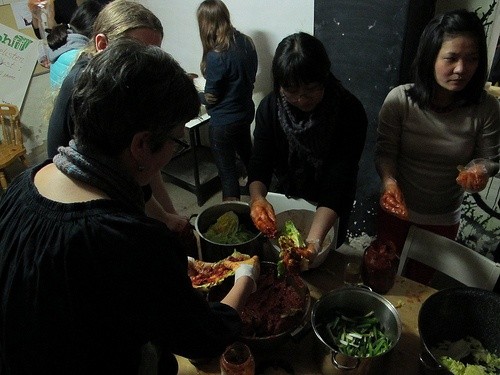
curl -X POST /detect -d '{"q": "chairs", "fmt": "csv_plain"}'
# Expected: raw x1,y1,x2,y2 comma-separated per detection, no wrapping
0,103,32,192
265,192,341,252
398,226,500,291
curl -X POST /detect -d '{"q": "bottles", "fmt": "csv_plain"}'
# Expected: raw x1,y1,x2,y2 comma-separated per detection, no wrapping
219,344,255,375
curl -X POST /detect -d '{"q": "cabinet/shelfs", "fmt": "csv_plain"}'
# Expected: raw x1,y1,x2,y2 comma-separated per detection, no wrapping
162,106,219,208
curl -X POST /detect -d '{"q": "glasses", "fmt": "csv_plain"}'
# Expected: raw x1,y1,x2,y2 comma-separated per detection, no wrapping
282,86,325,103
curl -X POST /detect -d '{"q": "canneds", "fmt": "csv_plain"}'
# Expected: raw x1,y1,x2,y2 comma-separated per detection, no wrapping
361,238,398,295
219,342,255,375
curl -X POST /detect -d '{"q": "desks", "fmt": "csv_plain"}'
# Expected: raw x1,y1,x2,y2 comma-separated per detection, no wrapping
174,241,442,375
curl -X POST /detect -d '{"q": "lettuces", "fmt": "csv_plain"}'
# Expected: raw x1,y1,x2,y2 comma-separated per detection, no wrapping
204,210,256,244
432,334,500,375
186,251,251,288
277,218,310,275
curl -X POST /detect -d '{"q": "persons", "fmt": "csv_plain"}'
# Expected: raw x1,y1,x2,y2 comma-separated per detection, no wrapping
0,40,260,375
47,0,104,98
248,33,368,272
375,9,500,285
196,0,258,201
47,0,199,269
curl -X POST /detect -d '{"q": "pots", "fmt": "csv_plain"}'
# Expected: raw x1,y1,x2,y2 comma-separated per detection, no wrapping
310,284,403,372
266,208,334,269
417,286,495,375
187,200,264,263
216,261,312,343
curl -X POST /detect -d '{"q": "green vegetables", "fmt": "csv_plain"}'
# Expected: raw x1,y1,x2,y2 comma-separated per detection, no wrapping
327,310,391,358
